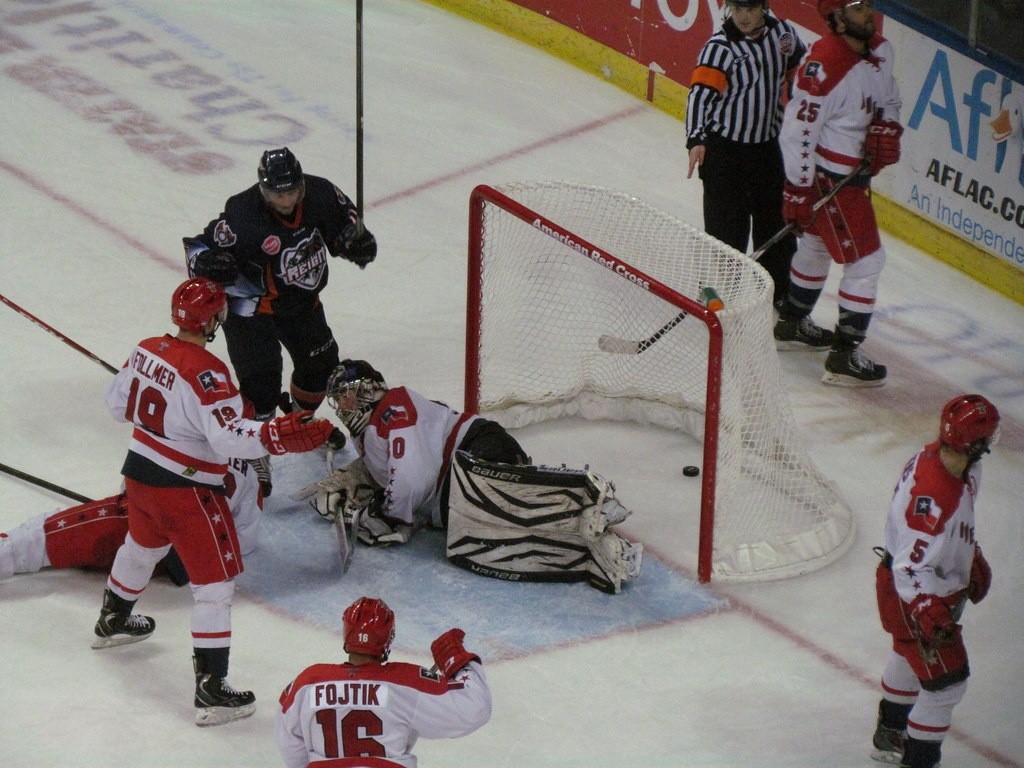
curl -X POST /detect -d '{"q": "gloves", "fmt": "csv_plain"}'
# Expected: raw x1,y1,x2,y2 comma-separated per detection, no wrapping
908,593,962,650
347,228,376,266
969,552,992,604
866,120,903,174
263,412,336,455
430,627,482,678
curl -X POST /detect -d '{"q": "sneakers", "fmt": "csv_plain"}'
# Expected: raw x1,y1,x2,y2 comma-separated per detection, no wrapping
621,542,643,579
822,343,887,387
91,587,158,648
192,655,256,727
248,456,272,505
593,502,632,532
315,429,348,453
774,318,836,351
871,722,942,768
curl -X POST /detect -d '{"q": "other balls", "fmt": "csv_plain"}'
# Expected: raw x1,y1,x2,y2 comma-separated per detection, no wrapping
683,466,700,476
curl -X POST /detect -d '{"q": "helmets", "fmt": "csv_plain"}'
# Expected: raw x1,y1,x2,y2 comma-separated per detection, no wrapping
940,393,1000,456
258,147,304,195
819,0,856,14
725,0,769,10
341,597,396,656
172,277,228,337
326,359,388,437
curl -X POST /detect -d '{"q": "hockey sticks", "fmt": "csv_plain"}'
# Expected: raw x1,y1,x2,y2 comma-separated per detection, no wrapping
355,1,366,267
0,463,95,505
598,157,868,355
923,592,971,663
0,295,120,376
324,447,350,575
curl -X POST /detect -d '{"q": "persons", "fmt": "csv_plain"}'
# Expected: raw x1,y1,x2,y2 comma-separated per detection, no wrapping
685,0,807,314
276,596,492,768
0,278,334,709
182,147,378,451
773,0,904,380
290,358,630,596
873,395,1001,768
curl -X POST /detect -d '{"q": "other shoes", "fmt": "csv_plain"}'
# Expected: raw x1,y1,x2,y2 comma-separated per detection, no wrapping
782,182,817,240
